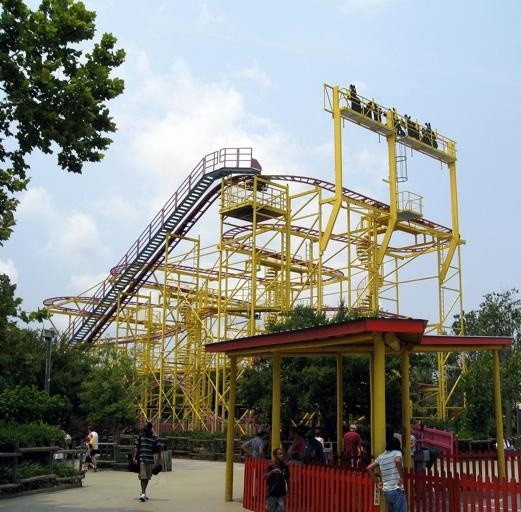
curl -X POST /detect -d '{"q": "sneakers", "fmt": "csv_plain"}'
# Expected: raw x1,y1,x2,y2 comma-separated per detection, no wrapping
139,492,150,502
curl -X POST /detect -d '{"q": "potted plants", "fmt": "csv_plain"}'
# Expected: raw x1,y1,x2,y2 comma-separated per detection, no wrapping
0,417,86,498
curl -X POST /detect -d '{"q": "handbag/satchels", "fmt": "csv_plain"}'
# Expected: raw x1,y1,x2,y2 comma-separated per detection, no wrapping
127,457,141,473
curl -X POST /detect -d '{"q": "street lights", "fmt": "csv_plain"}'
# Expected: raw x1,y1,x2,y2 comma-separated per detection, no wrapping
41,329,55,392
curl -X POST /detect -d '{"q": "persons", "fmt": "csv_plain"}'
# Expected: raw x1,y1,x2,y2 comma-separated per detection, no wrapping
122,425,130,443
240,421,417,468
365,438,408,512
132,421,161,501
262,448,290,511
496,433,516,452
55,425,100,472
